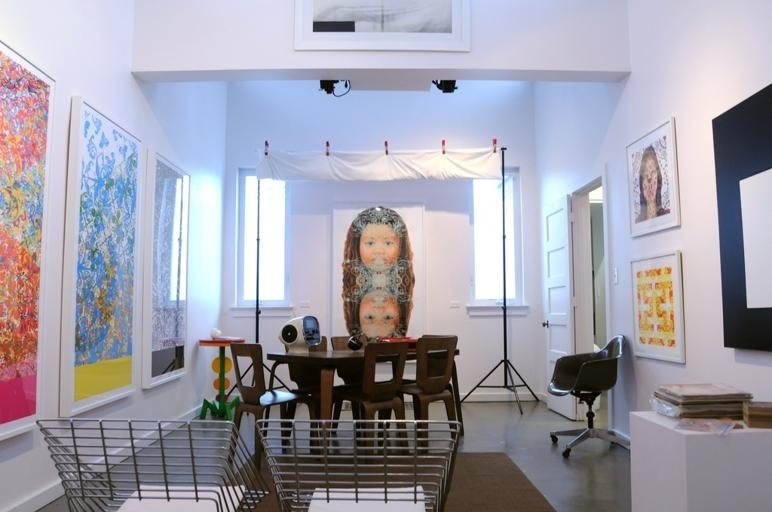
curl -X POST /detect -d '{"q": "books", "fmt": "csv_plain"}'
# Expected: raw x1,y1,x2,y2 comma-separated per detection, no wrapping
654,385,772,431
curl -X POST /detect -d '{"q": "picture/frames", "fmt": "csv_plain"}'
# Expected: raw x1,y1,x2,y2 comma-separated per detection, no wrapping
625,116,681,240
0,38,56,443
292,0,473,54
137,147,194,391
629,250,686,365
57,93,146,420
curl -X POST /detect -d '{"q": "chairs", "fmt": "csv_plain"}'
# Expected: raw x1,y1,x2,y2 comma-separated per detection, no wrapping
228,335,457,474
547,336,631,457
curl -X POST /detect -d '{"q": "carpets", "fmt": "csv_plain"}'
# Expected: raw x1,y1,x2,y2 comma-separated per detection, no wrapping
223,450,556,511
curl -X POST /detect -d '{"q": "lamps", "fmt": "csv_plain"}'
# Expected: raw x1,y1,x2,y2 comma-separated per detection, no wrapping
436,80,457,94
319,78,339,94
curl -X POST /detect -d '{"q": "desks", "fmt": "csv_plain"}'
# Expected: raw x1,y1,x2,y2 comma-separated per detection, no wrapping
266,348,460,464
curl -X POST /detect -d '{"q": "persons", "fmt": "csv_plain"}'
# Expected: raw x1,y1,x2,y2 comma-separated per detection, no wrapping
343,281,415,342
635,145,670,223
343,207,415,282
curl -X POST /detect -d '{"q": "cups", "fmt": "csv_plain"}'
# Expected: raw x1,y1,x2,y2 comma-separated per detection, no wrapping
347,337,363,350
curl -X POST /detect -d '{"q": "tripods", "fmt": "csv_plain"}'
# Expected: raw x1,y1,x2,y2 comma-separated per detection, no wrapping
460,149,540,415
225,180,292,416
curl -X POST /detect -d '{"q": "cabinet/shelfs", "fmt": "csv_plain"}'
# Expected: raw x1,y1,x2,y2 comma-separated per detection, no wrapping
629,410,772,512
